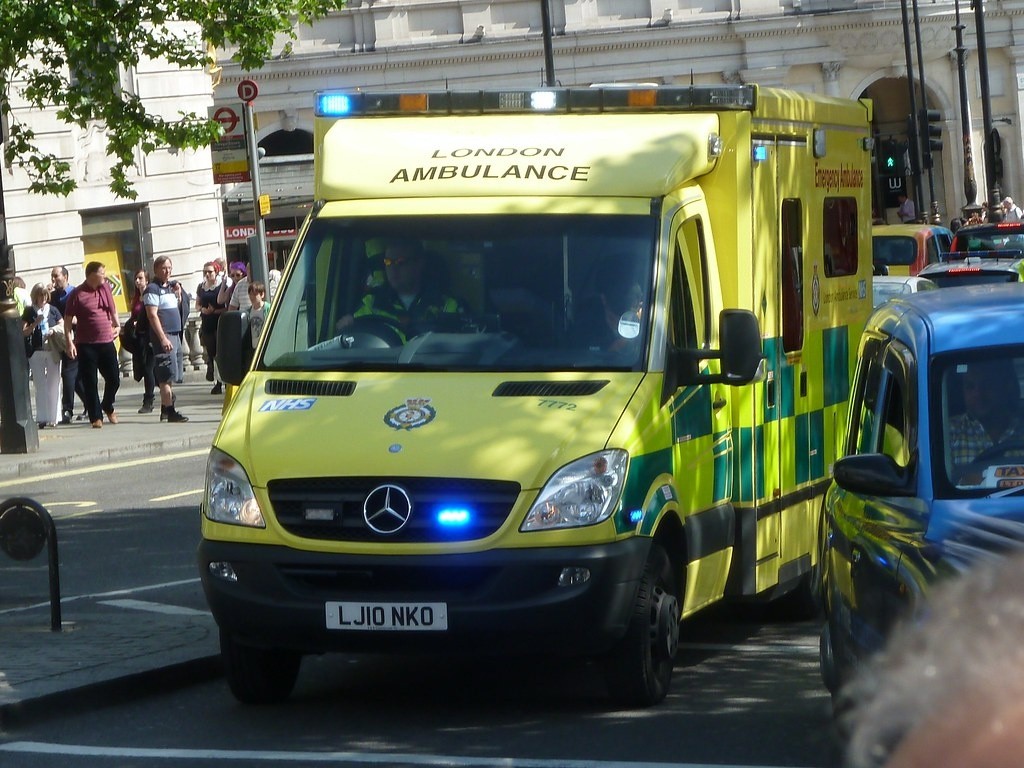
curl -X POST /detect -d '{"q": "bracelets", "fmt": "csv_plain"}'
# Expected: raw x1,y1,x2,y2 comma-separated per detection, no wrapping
30,324,34,329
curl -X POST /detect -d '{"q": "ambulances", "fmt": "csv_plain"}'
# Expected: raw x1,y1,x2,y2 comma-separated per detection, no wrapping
197,85,875,707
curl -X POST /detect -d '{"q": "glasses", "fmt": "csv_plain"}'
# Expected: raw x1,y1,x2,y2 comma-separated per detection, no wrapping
203,270,215,274
231,273,243,277
383,255,415,266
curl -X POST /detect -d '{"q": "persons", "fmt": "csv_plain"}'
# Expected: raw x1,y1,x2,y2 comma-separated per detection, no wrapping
22,256,190,429
848,557,1024,768
950,196,1024,234
947,363,1024,487
337,234,465,344
195,257,281,395
872,193,916,227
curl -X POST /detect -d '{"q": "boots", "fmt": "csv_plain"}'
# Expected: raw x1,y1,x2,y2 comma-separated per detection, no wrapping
139,394,155,413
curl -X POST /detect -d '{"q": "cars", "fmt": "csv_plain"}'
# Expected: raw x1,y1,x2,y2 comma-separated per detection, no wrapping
872,223,1024,318
810,282,1024,763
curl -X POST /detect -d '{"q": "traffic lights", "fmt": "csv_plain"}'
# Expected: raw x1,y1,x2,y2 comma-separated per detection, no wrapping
927,110,944,151
878,138,903,175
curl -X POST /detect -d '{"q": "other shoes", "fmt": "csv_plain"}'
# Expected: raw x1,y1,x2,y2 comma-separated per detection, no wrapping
211,380,222,394
58,419,72,425
101,403,118,424
77,409,90,421
92,419,102,428
206,366,214,381
161,405,188,422
